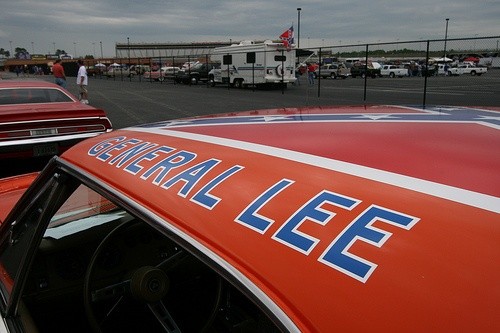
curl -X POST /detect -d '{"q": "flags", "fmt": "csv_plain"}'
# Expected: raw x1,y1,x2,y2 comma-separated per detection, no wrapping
279,26,294,49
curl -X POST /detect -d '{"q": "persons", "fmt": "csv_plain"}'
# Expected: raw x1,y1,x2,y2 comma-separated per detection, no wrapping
444,64,449,76
408,61,439,77
76,59,89,104
52,59,66,87
307,62,315,86
16,64,53,76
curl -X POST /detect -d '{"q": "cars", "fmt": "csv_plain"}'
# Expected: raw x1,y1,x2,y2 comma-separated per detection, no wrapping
1,105,500,333
88,63,137,77
0,79,115,159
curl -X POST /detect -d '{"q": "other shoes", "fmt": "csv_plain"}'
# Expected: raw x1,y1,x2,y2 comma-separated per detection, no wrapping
79,99,89,104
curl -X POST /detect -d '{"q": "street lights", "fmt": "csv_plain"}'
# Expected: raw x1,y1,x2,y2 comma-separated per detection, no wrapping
100,41,103,59
127,37,131,64
444,18,450,58
296,7,303,65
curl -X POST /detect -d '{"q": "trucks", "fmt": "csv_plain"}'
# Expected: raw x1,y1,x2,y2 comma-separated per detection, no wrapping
207,40,297,88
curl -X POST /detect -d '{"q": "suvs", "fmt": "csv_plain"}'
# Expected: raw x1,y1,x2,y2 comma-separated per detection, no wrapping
145,61,220,85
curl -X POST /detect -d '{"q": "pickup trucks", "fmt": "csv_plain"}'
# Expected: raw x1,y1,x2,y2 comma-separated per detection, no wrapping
350,60,381,78
432,61,489,75
311,64,351,77
380,65,410,78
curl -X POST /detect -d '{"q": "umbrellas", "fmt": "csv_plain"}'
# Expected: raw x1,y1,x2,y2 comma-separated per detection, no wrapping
95,63,120,66
